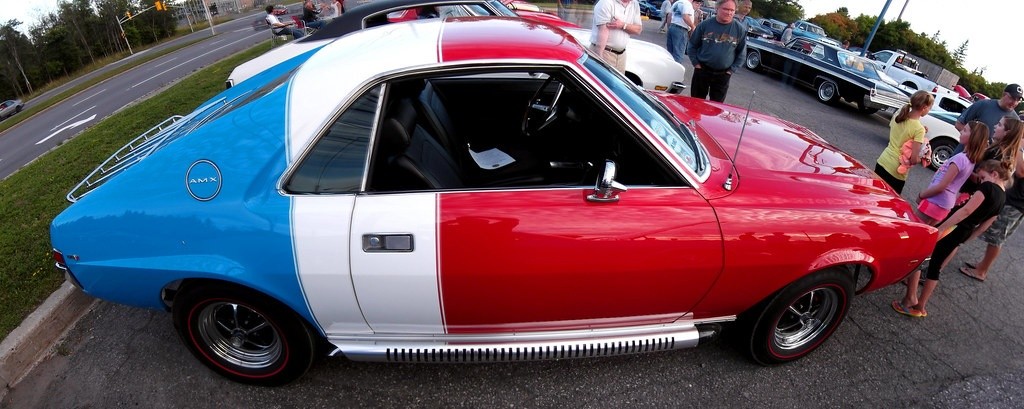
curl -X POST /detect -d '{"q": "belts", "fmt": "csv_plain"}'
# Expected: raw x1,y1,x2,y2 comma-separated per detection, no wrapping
591,43,625,54
676,25,688,31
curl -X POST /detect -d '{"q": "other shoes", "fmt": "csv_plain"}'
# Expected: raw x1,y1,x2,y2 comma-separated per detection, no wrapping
657,28,666,33
919,268,929,285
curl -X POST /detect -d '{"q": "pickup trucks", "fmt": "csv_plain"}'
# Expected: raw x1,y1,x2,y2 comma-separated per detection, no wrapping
852,49,976,129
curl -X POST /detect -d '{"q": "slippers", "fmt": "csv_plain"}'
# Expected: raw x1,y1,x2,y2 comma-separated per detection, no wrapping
892,300,922,317
959,267,984,282
922,310,927,316
965,261,977,268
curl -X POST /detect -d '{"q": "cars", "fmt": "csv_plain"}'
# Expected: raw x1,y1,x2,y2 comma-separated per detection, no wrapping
638,0,913,133
50,0,939,388
953,84,990,107
272,5,288,15
0,99,23,121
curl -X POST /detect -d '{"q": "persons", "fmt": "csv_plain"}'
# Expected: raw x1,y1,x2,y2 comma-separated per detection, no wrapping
687,0,747,103
901,120,989,288
265,5,304,39
956,84,1024,154
588,0,643,76
919,115,1024,285
777,22,796,45
867,52,876,60
302,0,346,31
891,158,1014,318
843,40,850,50
734,0,752,34
874,90,934,196
958,135,1024,282
656,0,706,65
558,0,576,21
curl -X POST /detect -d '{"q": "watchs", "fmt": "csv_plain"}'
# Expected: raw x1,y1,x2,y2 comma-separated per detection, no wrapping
623,23,627,30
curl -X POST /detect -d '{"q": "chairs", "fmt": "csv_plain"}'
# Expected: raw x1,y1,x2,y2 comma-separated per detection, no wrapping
381,96,464,189
295,14,315,37
410,78,535,183
265,17,294,49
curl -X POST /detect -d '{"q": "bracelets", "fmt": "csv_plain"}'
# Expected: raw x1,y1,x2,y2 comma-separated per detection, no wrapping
919,192,922,199
1020,148,1024,152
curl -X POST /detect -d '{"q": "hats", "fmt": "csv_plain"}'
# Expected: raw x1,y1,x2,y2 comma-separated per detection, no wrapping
1004,84,1024,101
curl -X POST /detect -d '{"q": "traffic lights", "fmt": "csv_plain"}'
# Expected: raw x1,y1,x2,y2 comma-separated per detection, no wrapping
120,33,125,40
127,13,133,20
163,4,167,10
